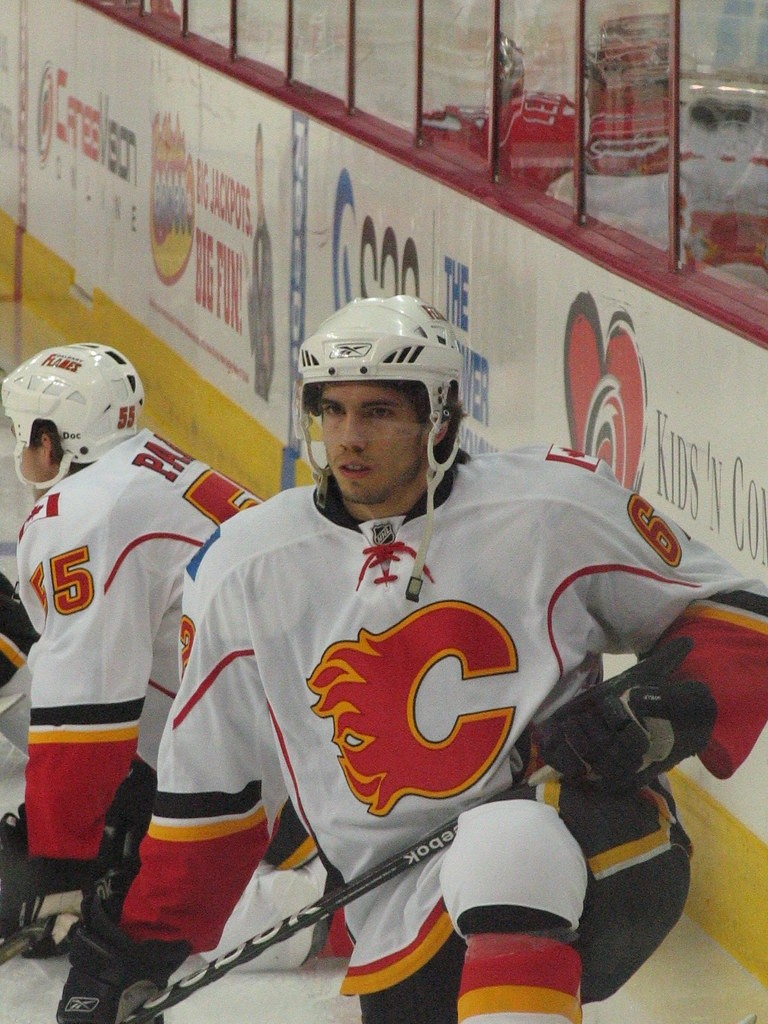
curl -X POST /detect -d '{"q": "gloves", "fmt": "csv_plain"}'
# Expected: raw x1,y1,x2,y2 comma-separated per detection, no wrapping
536,673,717,794
56,916,191,1024
3,859,114,959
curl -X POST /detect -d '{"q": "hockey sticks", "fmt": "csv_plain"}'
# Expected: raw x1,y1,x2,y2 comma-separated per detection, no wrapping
1,913,55,963
119,776,532,1024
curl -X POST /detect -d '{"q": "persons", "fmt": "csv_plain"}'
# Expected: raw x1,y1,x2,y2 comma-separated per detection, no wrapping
0,343,353,974
250,124,275,401
56,293,768,1024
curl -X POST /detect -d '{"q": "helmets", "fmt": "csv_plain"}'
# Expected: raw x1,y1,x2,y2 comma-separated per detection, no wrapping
0,342,144,463
297,295,464,475
